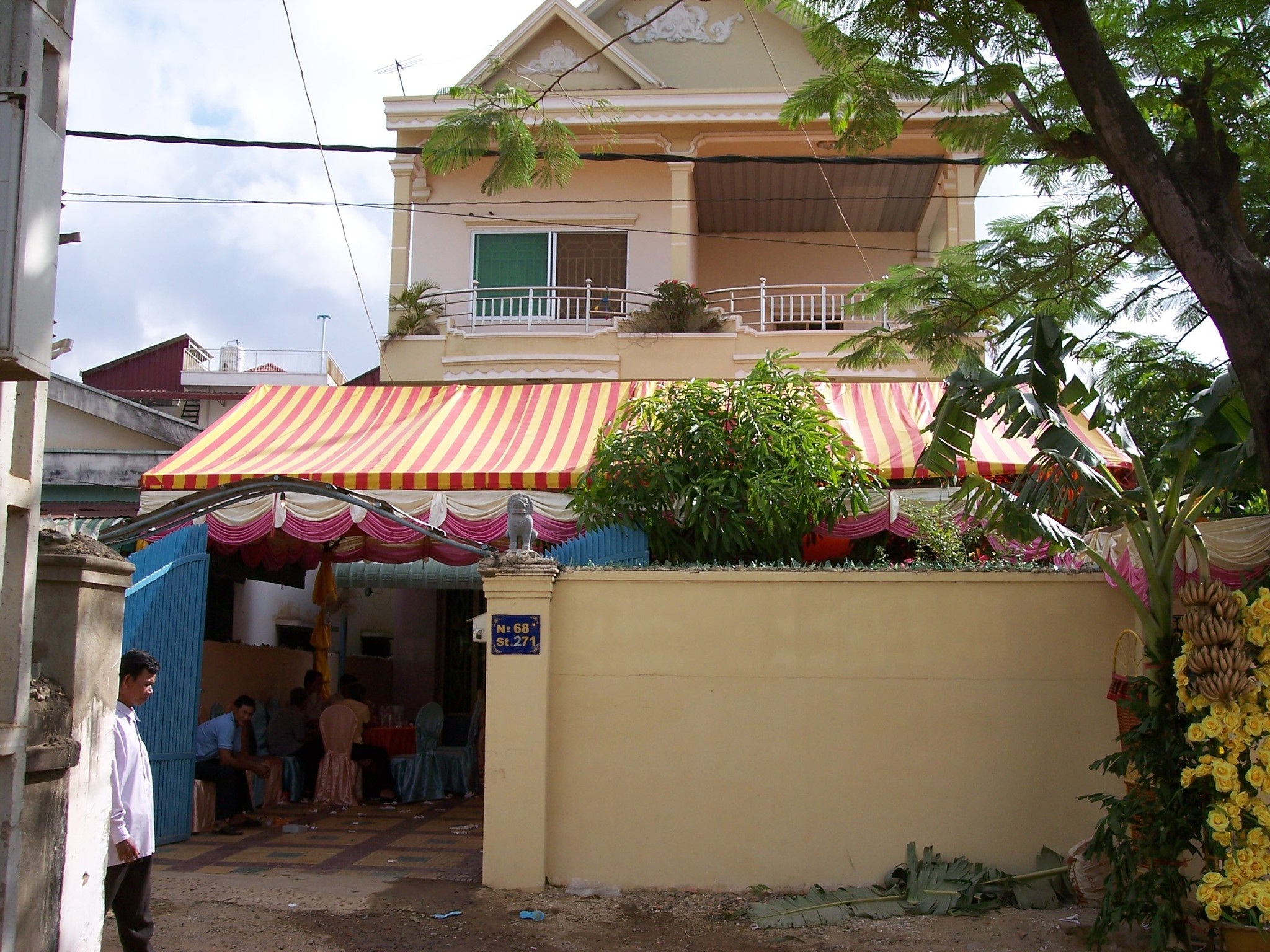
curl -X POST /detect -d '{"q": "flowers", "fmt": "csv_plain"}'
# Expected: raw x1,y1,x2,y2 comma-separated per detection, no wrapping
1173,586,1270,927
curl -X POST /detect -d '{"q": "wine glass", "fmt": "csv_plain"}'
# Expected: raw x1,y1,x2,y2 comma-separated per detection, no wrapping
393,705,404,728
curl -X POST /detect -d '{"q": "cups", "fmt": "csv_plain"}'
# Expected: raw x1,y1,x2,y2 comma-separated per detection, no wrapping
380,713,391,728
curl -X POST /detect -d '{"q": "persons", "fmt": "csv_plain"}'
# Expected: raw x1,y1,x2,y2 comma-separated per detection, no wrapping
194,695,273,836
265,669,401,803
104,648,162,952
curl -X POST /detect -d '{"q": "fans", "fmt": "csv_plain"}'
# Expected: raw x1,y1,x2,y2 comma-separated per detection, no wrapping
323,586,392,626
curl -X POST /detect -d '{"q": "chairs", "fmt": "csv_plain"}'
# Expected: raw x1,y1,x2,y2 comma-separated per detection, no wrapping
192,699,481,832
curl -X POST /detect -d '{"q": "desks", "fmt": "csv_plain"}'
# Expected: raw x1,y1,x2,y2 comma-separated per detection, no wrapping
362,726,416,759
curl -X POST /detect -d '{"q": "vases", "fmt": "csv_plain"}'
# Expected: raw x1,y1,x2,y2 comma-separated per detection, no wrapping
1222,916,1270,952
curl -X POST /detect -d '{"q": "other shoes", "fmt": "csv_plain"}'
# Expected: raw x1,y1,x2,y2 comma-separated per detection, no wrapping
380,795,400,804
299,793,310,803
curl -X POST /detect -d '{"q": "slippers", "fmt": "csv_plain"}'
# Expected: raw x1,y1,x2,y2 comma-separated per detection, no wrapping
230,817,263,827
212,825,243,835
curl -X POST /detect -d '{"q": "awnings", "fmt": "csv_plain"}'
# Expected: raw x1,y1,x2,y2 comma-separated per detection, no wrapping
129,382,1136,565
